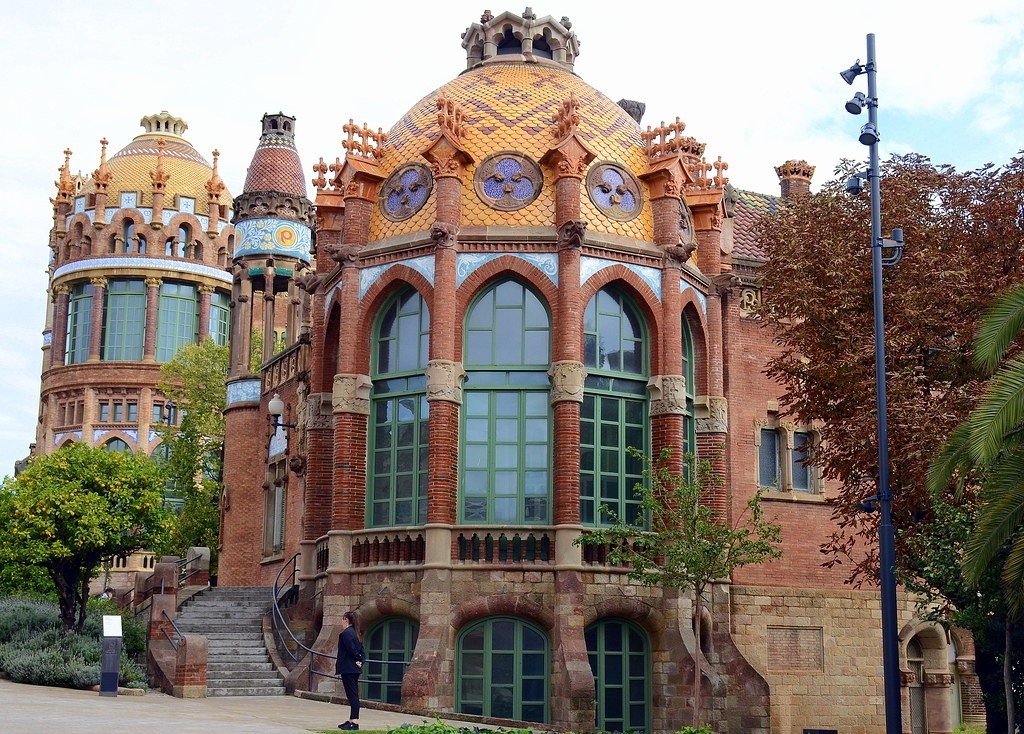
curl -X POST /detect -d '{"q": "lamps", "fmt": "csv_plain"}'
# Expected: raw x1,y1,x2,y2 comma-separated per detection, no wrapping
268,390,297,436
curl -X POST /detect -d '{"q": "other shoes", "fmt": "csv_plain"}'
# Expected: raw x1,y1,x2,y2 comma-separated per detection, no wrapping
338,721,359,730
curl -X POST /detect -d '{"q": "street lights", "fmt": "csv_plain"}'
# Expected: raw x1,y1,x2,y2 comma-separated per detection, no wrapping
835,29,911,734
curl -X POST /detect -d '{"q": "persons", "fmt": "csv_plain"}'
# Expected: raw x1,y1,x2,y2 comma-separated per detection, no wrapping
334,611,366,730
101,588,113,600
